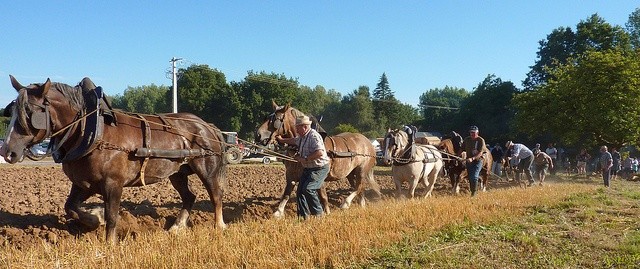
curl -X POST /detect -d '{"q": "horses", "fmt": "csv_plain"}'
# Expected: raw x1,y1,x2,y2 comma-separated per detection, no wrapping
384,126,443,198
441,136,493,194
256,98,381,219
4,74,228,244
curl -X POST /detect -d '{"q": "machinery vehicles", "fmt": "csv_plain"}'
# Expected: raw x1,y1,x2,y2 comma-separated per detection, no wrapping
220,132,244,165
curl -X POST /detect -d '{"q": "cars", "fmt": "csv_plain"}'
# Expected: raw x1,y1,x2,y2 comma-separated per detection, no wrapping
369,138,392,167
242,144,277,164
30,140,50,158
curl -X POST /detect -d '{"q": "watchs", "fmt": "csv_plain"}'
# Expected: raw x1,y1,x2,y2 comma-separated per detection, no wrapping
472,157,474,161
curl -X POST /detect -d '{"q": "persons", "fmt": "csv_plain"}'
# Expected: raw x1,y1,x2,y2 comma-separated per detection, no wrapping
486,143,493,151
624,153,633,179
455,126,488,197
599,146,613,188
611,147,621,180
505,140,536,188
490,141,505,179
275,116,332,220
633,156,639,175
531,148,553,186
545,141,559,176
534,143,541,155
562,146,592,179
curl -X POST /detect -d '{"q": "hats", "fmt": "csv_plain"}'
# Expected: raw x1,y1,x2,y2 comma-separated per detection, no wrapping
532,148,541,155
505,140,512,149
292,116,312,125
470,126,478,132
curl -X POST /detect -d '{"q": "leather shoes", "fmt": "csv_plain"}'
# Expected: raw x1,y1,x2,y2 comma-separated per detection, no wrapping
527,181,536,186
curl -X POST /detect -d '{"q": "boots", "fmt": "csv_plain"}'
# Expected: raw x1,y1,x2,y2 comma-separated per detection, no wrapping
470,181,477,197
